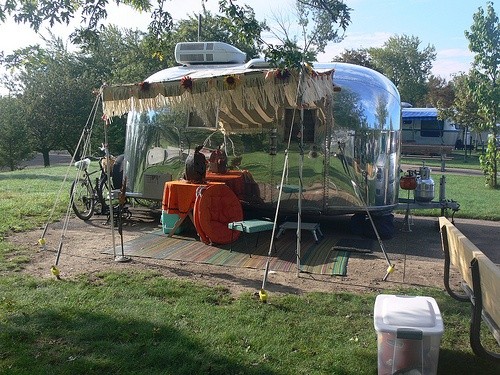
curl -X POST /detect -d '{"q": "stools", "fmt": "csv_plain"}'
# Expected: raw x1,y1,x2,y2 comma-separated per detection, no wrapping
228,218,276,258
275,222,324,245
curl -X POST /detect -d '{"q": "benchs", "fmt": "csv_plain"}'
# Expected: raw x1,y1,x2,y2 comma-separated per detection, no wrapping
438,215,500,362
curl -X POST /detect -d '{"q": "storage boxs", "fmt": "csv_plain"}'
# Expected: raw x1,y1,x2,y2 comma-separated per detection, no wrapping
374,294,444,375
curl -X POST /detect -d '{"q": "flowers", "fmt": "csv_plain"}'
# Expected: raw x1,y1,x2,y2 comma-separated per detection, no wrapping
180,76,193,89
140,80,151,92
275,67,291,81
223,74,239,87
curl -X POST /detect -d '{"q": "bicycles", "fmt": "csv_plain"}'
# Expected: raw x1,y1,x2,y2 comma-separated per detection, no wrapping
70,141,127,222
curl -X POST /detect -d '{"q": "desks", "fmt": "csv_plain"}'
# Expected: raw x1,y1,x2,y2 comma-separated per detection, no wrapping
205,168,263,210
162,182,244,245
277,184,308,207
